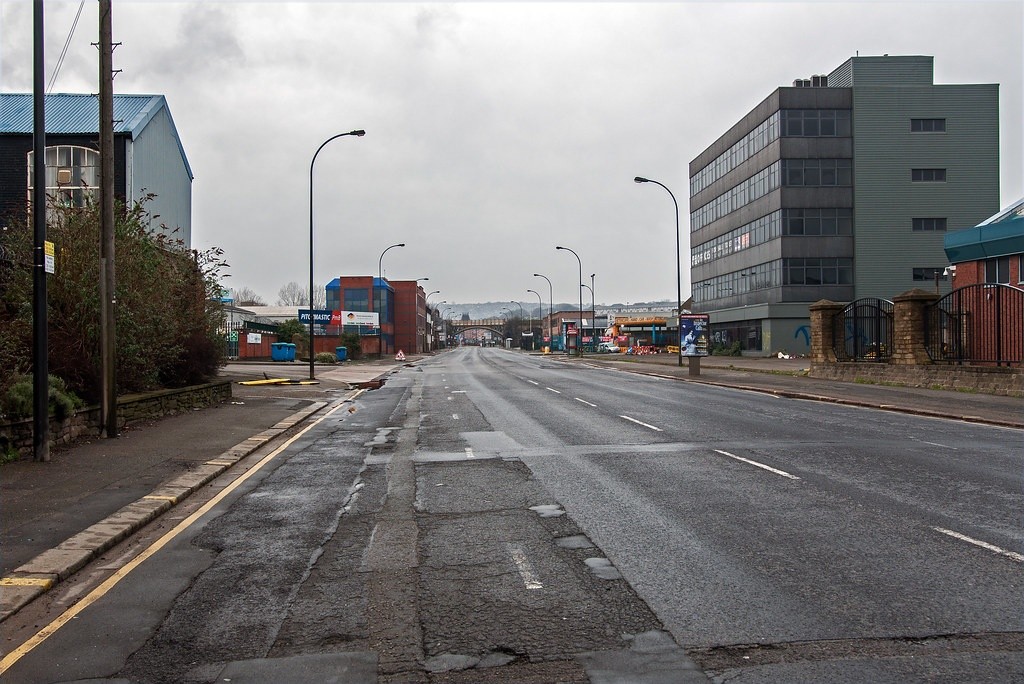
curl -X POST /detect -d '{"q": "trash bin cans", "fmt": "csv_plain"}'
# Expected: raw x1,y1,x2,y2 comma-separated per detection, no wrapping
335,346,347,361
271,342,288,362
287,343,296,363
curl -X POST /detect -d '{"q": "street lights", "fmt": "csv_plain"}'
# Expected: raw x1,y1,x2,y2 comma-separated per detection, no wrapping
441,308,451,332
454,314,461,319
581,274,596,348
450,316,458,348
426,291,439,352
409,278,429,356
634,176,683,366
434,301,446,350
446,311,455,322
503,307,513,319
511,301,522,349
500,312,508,321
527,289,542,348
309,130,366,381
556,246,583,357
534,273,553,353
378,243,405,357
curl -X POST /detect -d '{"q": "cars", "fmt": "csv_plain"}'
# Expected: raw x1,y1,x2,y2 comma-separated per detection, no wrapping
597,342,620,353
